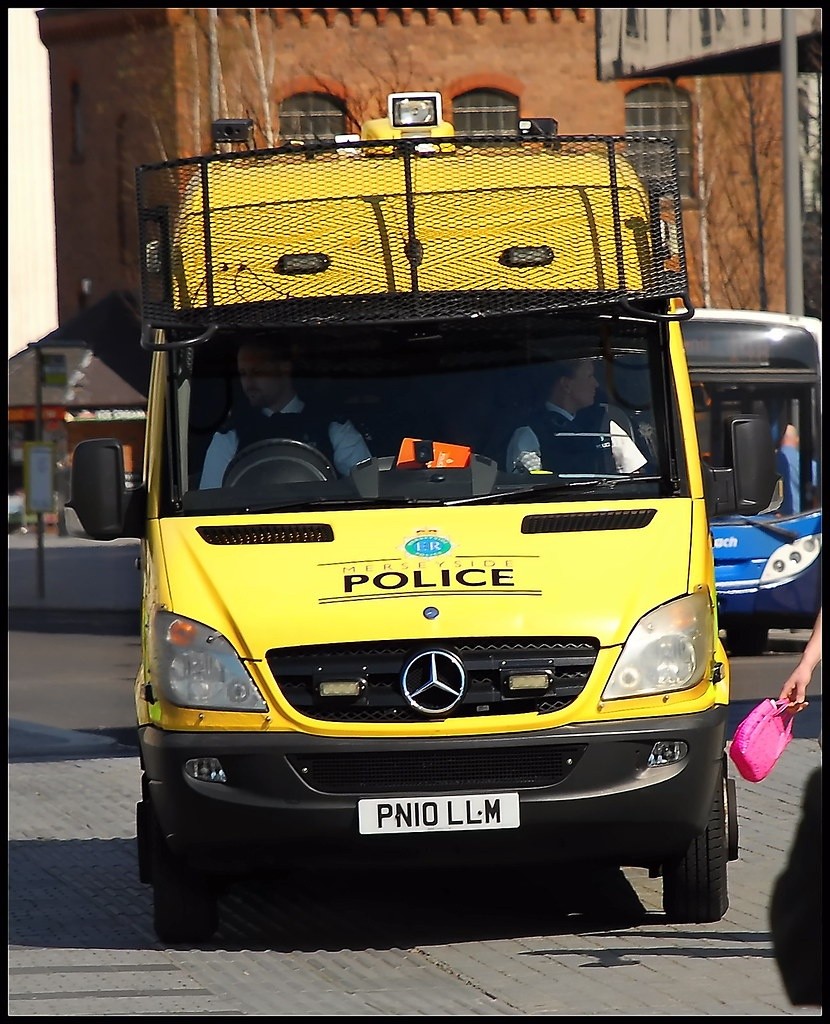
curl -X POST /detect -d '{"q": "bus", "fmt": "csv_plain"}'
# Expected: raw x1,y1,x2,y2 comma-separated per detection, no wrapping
595,308,828,655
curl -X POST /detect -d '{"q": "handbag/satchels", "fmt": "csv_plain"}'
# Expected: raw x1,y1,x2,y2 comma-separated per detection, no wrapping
730,696,795,784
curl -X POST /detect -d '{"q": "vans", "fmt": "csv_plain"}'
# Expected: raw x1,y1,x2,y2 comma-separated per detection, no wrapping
63,92,743,945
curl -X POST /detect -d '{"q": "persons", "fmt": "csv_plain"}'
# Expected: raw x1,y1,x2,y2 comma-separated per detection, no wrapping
505,341,647,474
780,604,822,715
771,424,817,513
198,332,373,489
